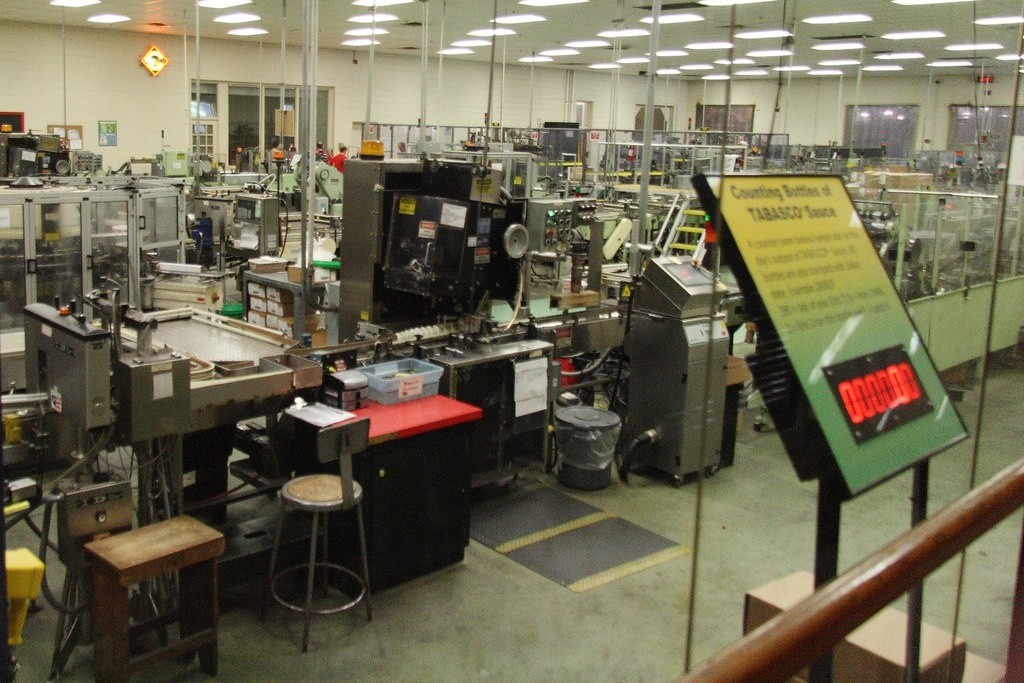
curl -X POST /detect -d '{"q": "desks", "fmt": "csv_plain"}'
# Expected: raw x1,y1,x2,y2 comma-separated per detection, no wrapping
243,270,333,346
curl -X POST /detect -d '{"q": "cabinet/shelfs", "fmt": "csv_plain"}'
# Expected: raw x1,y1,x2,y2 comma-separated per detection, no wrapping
331,422,483,594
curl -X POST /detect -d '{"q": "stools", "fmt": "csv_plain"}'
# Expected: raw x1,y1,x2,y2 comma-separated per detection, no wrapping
260,418,373,654
84,515,225,683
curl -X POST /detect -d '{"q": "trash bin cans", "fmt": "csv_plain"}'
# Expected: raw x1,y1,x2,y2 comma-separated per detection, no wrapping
553,405,623,491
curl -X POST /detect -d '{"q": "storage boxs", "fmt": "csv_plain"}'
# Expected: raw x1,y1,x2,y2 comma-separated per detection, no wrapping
288,265,336,284
249,257,287,274
739,572,1007,683
845,165,933,227
356,358,444,405
249,283,327,348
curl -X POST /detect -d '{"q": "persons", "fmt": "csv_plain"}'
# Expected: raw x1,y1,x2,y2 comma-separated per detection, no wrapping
270,141,280,171
287,142,347,173
